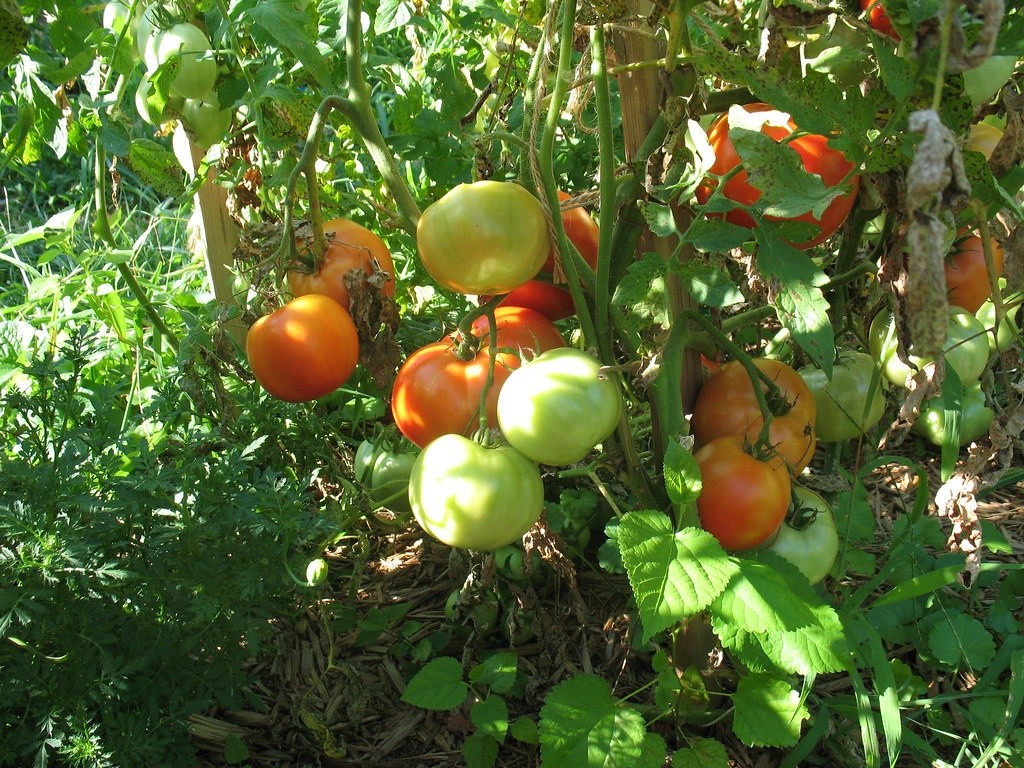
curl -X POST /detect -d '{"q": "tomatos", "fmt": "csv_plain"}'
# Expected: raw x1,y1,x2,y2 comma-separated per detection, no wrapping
246,103,1004,645
859,0,911,42
103,1,234,150
